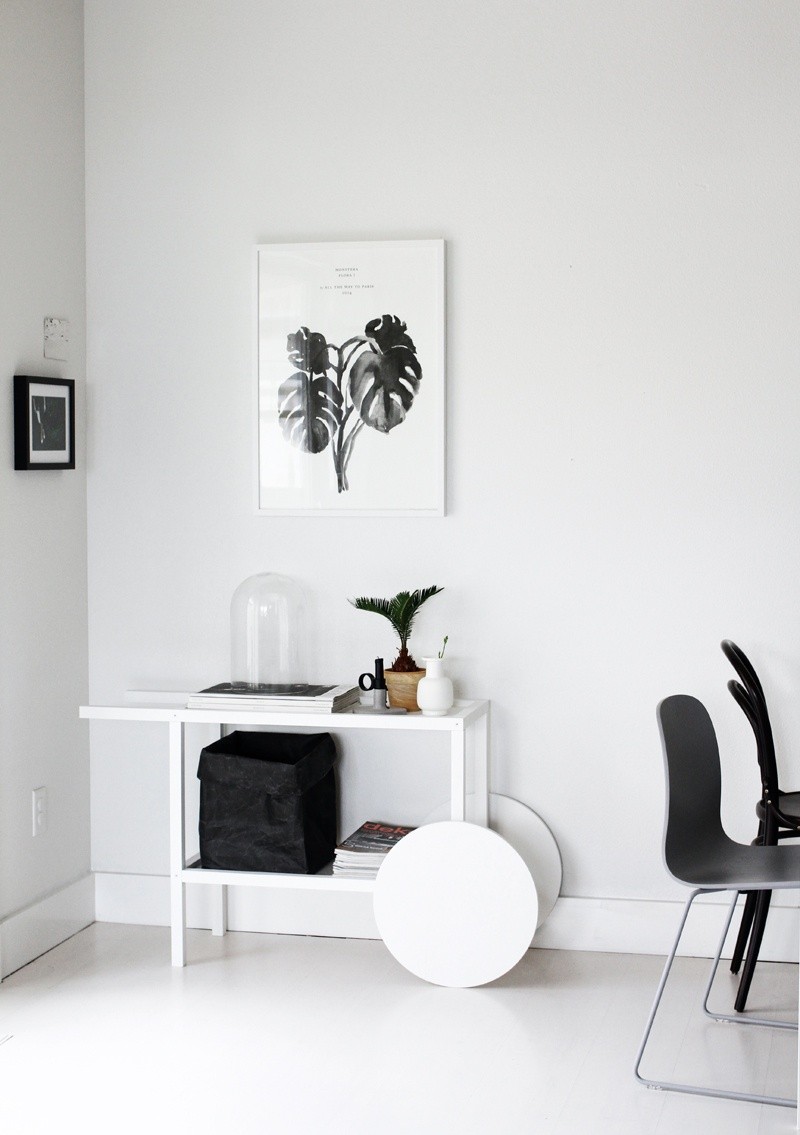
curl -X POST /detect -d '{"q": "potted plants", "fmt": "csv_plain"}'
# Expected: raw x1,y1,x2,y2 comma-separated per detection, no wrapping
348,584,446,712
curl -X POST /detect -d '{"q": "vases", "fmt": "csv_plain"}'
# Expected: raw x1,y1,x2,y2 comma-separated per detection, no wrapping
417,656,453,716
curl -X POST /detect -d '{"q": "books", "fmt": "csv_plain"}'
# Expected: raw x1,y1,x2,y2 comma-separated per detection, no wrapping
333,820,419,879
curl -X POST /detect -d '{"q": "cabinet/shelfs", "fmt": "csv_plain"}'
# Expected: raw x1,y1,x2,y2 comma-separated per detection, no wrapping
78,692,492,969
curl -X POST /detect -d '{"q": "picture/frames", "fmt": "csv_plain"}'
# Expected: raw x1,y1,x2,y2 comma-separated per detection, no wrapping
254,239,450,520
14,375,76,471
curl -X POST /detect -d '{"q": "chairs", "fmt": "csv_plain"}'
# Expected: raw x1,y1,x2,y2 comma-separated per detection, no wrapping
721,638,800,1013
633,694,799,1108
